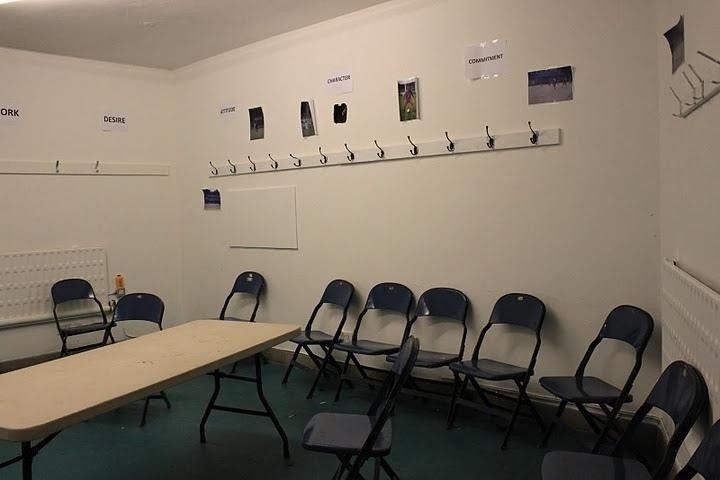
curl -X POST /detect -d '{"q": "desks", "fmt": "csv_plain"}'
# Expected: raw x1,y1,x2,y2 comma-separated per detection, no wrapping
0,320,301,480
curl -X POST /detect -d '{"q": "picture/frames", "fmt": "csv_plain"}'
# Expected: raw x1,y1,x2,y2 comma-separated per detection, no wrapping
396,77,421,124
246,103,268,142
298,99,318,139
526,64,576,107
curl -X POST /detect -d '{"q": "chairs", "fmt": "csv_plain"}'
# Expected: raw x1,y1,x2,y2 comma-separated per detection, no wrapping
83,292,171,427
197,271,269,373
51,278,117,357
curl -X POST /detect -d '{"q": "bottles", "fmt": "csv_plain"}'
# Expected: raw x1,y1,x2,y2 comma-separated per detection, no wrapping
115,274,125,296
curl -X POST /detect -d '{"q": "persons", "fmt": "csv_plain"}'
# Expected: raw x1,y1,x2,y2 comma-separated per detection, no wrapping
334,103,347,122
402,85,415,113
301,117,312,130
562,79,568,88
552,76,558,89
254,112,259,132
